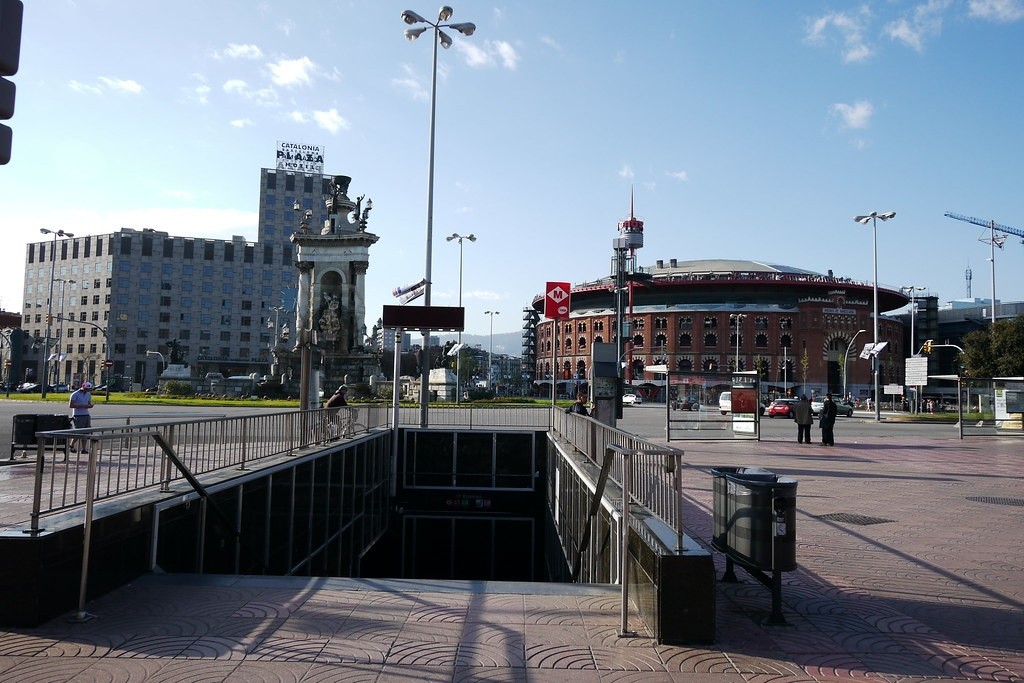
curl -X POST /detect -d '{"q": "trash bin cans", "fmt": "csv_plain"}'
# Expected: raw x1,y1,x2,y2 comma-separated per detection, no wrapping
9,414,71,463
725,471,799,572
709,465,774,552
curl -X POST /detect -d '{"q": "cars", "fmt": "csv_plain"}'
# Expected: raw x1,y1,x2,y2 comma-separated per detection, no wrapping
94,383,124,393
0,381,68,393
671,395,699,411
623,394,642,405
143,383,158,392
768,398,800,418
811,396,853,417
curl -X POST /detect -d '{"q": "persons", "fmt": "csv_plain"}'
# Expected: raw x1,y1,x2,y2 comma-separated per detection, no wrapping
818,393,837,446
792,395,814,444
866,398,872,412
900,397,947,414
69,381,94,454
570,390,595,418
323,384,360,427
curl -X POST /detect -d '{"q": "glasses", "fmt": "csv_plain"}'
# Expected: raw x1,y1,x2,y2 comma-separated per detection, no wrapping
345,390,347,392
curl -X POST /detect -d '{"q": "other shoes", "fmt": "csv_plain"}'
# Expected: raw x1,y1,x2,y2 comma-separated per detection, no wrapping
819,443,833,446
81,449,88,454
69,444,77,453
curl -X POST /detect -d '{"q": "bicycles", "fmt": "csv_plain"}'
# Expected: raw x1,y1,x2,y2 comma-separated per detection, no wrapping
312,408,368,442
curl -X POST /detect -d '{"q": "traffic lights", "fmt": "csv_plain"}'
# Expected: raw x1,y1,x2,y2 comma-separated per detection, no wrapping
100,360,105,372
923,343,927,353
46,314,52,324
927,341,934,354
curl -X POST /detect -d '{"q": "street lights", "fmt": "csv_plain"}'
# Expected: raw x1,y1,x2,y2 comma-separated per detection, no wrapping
843,330,866,398
40,228,75,398
901,285,926,412
609,285,629,377
269,306,285,364
484,311,500,390
730,314,748,383
978,220,1008,321
401,5,478,428
854,211,897,420
447,232,477,405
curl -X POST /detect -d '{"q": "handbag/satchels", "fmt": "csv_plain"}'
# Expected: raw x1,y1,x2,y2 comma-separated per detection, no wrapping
819,409,829,421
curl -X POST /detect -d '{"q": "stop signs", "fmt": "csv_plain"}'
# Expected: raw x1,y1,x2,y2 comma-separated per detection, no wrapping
105,359,113,367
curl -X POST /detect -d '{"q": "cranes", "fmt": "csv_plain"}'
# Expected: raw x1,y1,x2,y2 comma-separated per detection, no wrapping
944,210,1024,244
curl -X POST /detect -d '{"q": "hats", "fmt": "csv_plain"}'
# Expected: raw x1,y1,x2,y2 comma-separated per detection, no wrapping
339,385,347,391
82,382,92,389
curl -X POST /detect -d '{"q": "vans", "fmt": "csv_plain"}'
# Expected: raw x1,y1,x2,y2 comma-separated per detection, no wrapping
718,392,765,416
